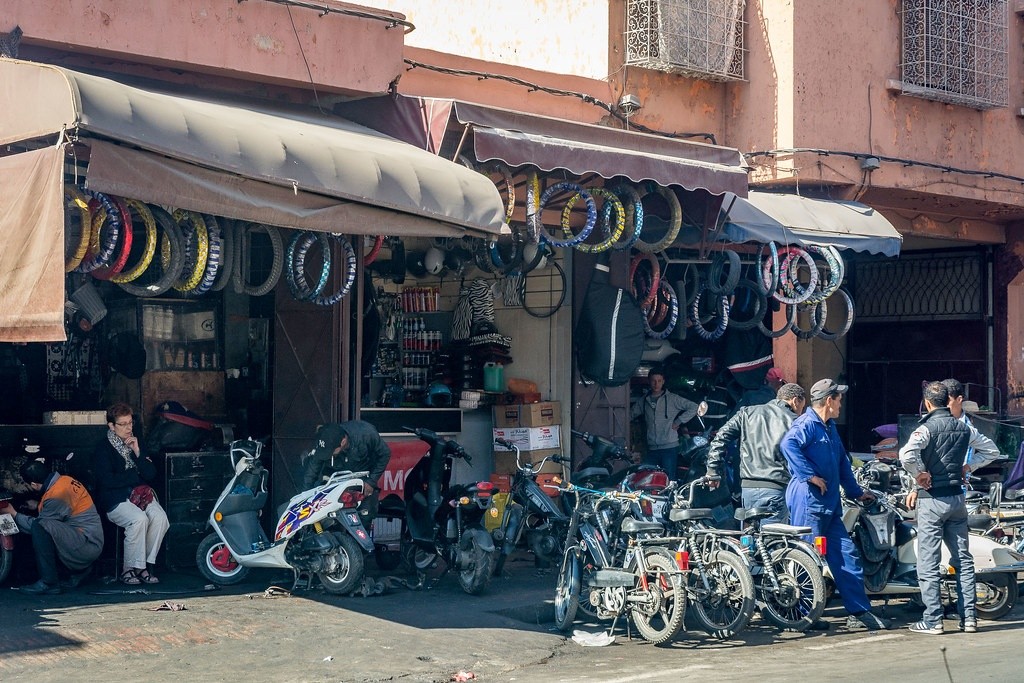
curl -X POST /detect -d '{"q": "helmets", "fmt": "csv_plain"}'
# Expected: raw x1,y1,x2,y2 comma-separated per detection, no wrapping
423,380,451,407
523,241,551,271
408,248,428,279
425,248,447,276
448,247,475,275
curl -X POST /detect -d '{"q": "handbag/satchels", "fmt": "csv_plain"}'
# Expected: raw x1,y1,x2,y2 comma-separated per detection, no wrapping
130,483,154,509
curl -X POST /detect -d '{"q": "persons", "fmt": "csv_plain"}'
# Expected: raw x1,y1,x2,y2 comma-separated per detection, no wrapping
302,420,391,578
898,380,1000,633
90,403,170,584
630,368,699,481
705,384,830,630
905,378,976,618
0,461,104,595
727,368,787,494
780,379,892,630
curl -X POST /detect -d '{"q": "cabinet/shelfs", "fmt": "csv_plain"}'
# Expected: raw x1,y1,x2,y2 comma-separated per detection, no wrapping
364,310,454,404
103,299,219,373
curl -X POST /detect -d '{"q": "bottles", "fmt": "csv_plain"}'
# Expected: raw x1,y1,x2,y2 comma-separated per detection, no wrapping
144,307,153,337
154,308,163,339
403,316,442,350
401,354,438,408
398,285,439,313
483,361,504,392
163,309,174,339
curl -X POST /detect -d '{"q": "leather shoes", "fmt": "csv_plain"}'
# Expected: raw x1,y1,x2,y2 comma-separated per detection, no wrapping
63,566,89,587
18,578,64,595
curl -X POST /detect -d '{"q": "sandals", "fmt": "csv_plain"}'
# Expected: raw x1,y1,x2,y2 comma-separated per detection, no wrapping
134,567,159,583
119,569,140,585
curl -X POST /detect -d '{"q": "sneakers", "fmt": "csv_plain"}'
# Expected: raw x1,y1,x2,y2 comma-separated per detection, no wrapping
957,619,977,632
909,619,944,635
808,617,830,631
845,611,892,631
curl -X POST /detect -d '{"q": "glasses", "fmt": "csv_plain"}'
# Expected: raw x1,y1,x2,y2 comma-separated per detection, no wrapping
109,418,135,429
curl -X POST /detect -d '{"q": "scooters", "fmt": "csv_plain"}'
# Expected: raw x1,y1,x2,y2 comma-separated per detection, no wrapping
490,401,834,647
822,453,1024,620
399,424,494,595
194,436,377,596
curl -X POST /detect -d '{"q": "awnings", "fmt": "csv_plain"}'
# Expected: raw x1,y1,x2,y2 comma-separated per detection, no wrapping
709,192,902,258
729,354,773,372
335,94,748,200
0,56,512,343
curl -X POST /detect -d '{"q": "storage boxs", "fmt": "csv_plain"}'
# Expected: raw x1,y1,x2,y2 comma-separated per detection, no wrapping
178,311,214,338
486,391,560,531
42,410,107,426
162,454,228,570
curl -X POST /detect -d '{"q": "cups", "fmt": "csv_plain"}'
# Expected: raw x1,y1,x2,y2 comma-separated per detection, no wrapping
232,368,240,378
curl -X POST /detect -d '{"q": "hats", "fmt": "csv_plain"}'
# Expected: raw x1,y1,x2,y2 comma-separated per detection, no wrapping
810,378,848,400
315,422,346,461
767,368,785,383
